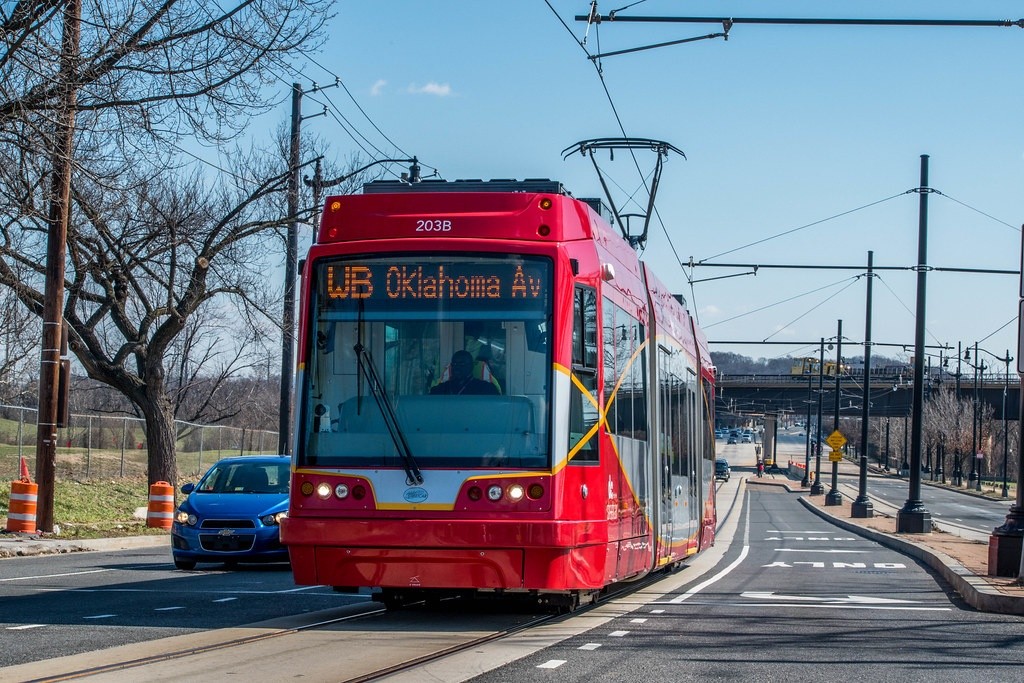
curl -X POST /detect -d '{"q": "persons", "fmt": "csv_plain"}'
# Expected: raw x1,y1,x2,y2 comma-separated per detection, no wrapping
272,470,290,494
842,356,846,364
756,459,764,478
429,349,502,396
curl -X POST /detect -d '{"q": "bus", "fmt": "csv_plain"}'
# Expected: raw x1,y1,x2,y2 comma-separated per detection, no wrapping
285,177,718,616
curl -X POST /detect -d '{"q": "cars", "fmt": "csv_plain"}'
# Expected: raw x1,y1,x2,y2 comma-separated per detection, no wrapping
713,424,764,446
715,459,731,482
170,454,291,571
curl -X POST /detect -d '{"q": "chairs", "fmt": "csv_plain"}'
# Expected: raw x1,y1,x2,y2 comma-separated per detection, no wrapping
246,465,288,493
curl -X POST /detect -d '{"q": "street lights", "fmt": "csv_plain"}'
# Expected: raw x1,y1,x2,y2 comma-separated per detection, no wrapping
963,345,1009,497
943,355,983,492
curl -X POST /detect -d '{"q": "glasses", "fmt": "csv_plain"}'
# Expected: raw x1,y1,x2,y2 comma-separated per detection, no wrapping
450,362,471,367
